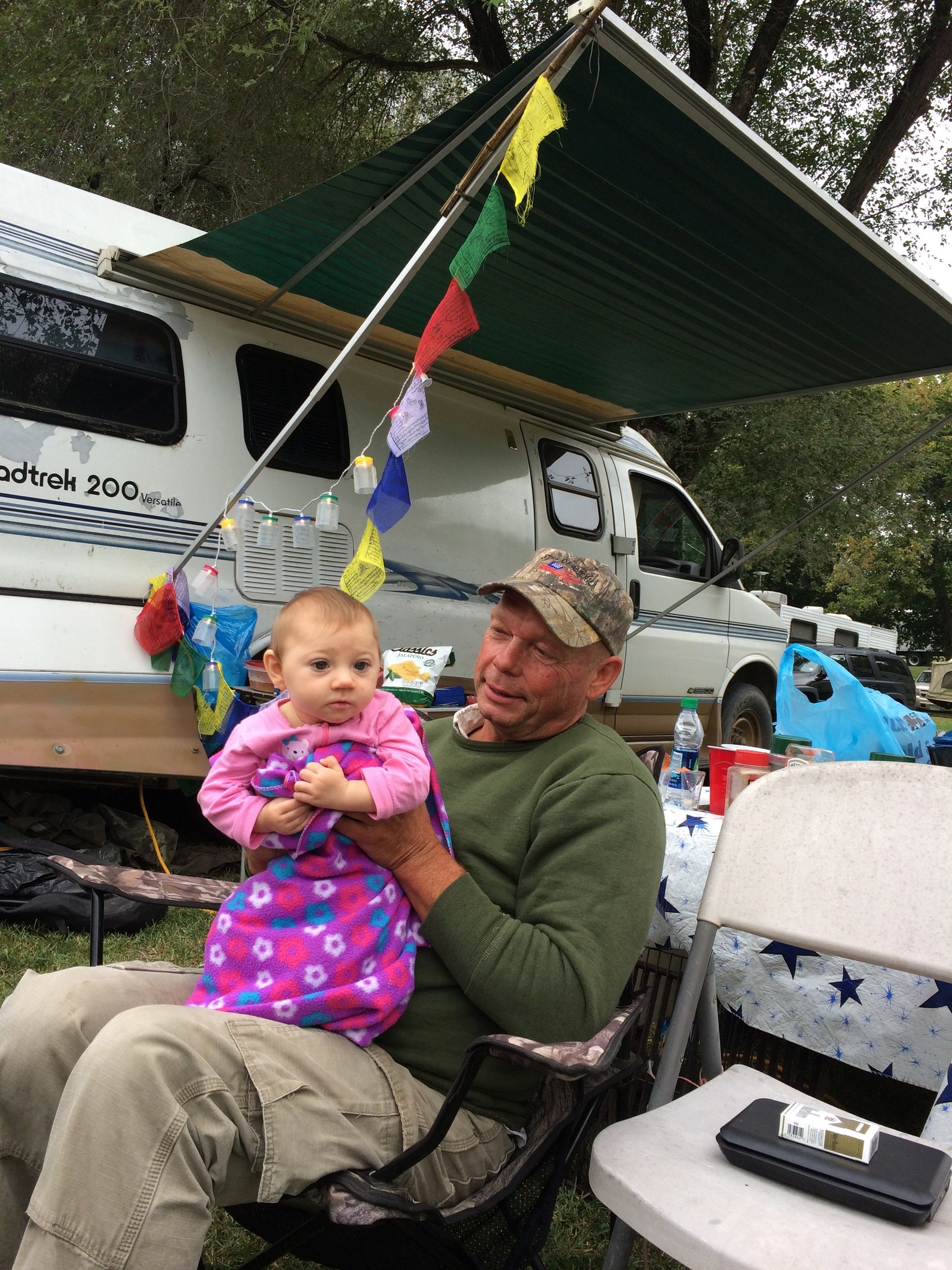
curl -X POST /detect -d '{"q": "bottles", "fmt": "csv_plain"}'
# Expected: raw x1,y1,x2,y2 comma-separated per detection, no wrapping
389,404,399,424
353,456,378,495
784,743,835,769
868,752,915,763
292,516,313,549
257,516,281,550
189,500,254,691
314,494,339,530
664,697,704,807
768,735,812,772
724,750,771,816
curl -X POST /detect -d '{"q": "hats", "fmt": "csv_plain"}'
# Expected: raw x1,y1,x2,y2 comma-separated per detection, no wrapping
477,547,635,658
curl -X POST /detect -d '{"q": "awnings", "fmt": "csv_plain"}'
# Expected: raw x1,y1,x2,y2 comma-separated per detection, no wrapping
96,1,952,454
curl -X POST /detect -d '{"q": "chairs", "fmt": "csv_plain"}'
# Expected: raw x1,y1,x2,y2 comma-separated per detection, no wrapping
590,761,952,1270
40,854,650,1270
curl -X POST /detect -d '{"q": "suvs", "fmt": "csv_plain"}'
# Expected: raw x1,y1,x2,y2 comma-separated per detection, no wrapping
789,640,916,711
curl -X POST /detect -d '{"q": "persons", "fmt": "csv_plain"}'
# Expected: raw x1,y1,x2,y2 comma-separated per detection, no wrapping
182,586,454,1044
0,546,667,1270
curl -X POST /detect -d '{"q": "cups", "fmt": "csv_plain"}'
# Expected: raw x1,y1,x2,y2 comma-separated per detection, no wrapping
657,767,672,806
707,743,770,816
681,771,705,811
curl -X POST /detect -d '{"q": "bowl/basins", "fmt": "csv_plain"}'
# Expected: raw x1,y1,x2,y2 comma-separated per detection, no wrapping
243,660,274,694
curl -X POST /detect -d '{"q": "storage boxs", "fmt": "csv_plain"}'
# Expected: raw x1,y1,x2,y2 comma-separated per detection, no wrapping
778,1101,880,1164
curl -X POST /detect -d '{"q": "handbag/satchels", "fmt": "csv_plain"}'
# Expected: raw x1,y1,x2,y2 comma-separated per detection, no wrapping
771,643,936,764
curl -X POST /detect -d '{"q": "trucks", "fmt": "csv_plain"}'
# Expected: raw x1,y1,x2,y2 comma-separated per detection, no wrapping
0,0,952,797
750,590,898,655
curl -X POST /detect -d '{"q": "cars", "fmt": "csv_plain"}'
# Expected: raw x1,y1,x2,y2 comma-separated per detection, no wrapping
913,669,952,714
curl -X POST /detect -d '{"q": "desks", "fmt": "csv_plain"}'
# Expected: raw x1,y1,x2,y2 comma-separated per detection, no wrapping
623,786,952,1152
231,686,465,723
